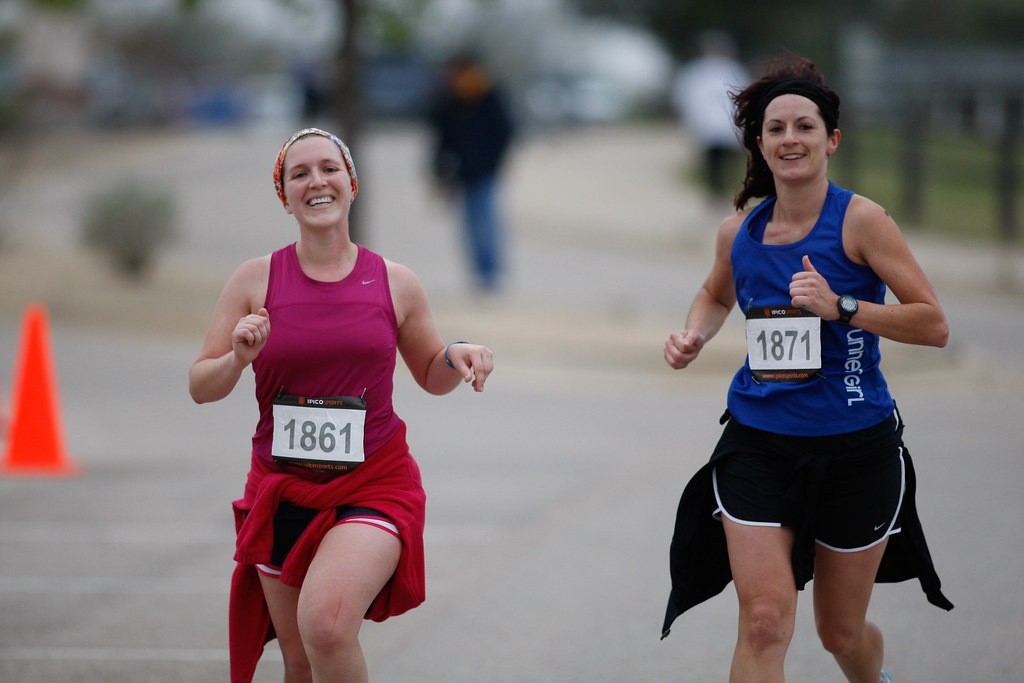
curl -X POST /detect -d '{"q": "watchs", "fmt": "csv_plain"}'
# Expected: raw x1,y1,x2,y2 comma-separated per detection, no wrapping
837,295,858,324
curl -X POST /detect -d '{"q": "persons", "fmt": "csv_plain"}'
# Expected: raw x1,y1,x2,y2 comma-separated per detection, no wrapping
430,53,515,298
189,126,494,683
661,58,955,683
675,30,753,199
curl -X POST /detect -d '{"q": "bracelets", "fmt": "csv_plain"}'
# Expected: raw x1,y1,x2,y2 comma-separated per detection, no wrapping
445,341,469,369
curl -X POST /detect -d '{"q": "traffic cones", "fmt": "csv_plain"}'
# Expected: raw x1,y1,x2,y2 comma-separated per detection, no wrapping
0,302,84,477
